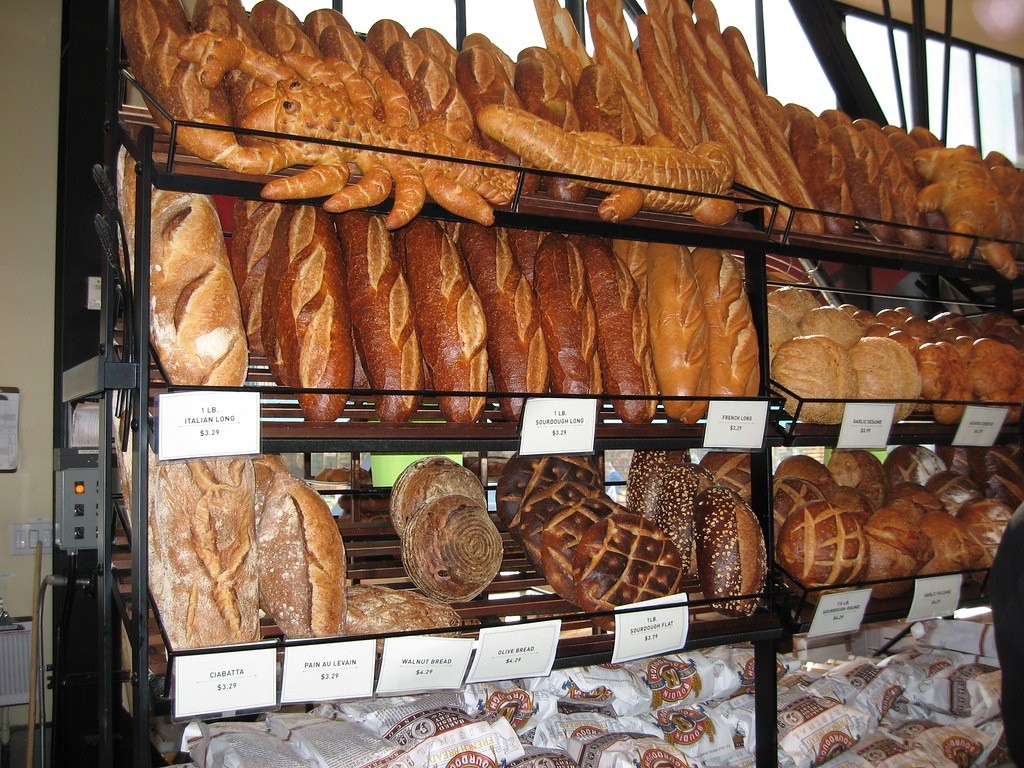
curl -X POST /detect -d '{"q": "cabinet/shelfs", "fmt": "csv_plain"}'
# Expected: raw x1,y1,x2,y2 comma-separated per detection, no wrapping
92,68,1024,768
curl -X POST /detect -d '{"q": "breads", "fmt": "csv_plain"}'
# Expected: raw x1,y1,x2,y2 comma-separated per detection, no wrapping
769,96,1024,593
118,0,823,648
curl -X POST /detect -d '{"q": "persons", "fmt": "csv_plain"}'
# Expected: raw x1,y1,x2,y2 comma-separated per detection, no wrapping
605,461,623,501
